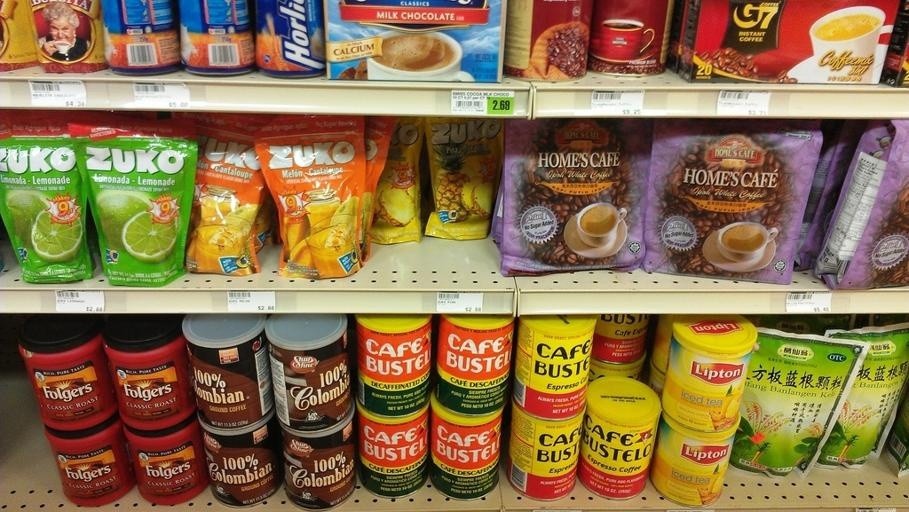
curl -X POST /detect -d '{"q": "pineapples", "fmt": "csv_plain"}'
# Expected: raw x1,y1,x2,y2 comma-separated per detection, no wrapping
373,144,492,228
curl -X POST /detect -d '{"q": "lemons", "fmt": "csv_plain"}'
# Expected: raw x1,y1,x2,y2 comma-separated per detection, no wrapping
4,190,179,263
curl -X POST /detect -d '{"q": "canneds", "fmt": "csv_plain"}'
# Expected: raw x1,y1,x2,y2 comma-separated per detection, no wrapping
100,0,327,79
182,315,758,509
586,0,676,77
505,0,594,83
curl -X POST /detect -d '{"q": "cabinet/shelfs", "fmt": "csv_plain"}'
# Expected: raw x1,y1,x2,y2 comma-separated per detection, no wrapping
0,0,909,512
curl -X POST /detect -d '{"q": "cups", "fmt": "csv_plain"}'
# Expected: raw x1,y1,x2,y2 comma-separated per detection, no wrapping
588,38,660,62
576,202,628,249
601,18,656,61
718,220,781,263
365,27,477,83
810,3,894,83
701,226,778,276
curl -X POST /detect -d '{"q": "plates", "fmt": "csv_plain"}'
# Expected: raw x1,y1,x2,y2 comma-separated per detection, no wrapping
783,42,890,86
563,209,629,259
